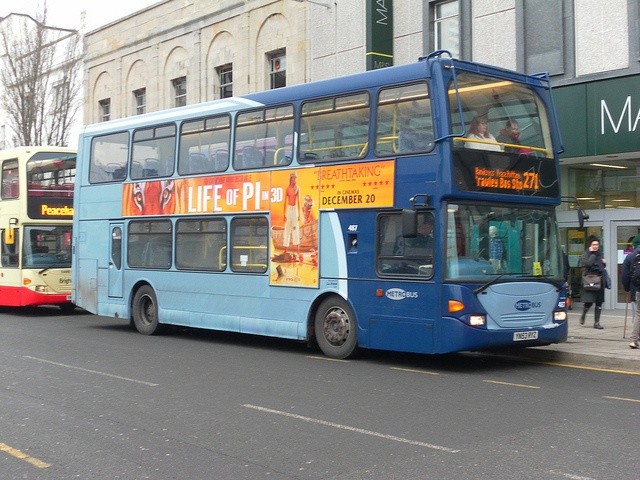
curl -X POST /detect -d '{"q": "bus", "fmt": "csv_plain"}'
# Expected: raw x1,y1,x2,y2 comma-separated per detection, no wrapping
65,49,572,360
0,146,79,312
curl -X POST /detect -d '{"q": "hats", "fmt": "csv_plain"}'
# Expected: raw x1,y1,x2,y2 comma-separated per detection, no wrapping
587,235,600,247
632,234,640,246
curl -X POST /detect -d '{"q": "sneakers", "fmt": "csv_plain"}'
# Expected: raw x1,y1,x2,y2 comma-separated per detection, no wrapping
629,342,640,348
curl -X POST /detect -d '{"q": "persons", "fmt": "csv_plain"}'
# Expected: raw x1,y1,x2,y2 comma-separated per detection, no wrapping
489,226,504,270
621,235,640,349
465,115,503,152
578,234,607,329
410,215,434,247
496,120,525,154
283,174,301,255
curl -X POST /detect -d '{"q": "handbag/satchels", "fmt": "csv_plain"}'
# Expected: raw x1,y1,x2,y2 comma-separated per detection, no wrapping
583,267,602,290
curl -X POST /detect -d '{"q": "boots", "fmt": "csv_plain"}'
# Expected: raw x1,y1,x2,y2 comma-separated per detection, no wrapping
580,308,588,324
594,306,604,329
